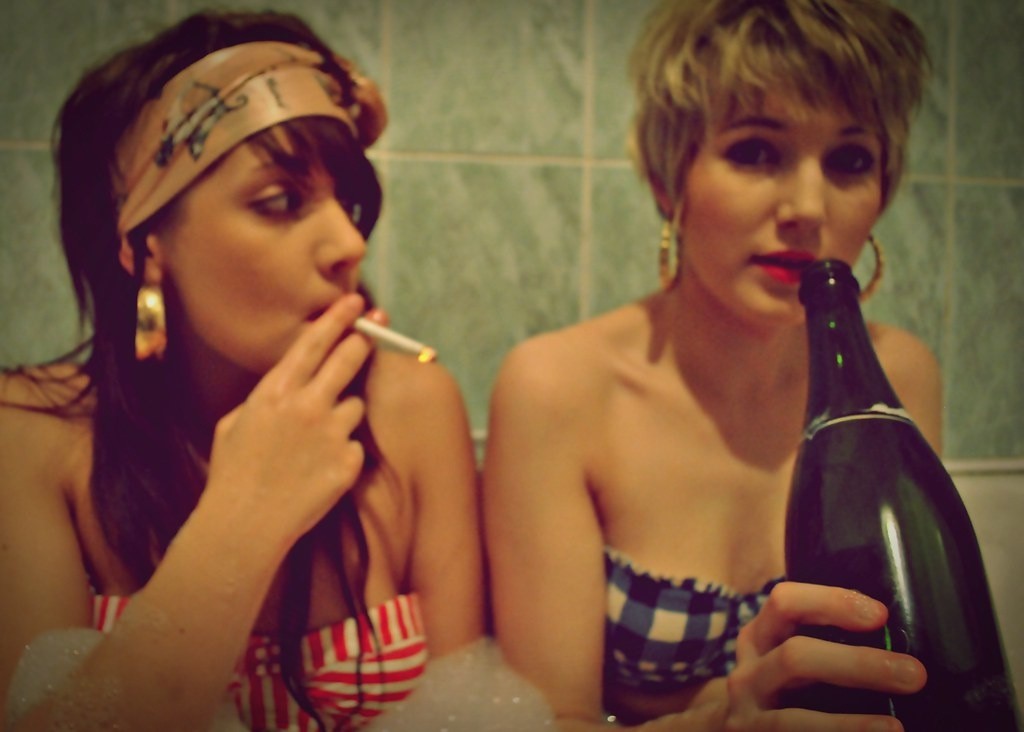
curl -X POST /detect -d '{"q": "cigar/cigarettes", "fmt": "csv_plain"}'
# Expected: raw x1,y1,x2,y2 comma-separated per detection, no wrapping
349,317,439,364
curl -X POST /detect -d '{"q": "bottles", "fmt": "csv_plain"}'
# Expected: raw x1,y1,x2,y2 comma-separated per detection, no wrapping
778,258,1019,732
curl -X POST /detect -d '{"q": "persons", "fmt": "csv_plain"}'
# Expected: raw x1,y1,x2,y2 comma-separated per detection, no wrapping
0,10,489,732
478,0,941,732
